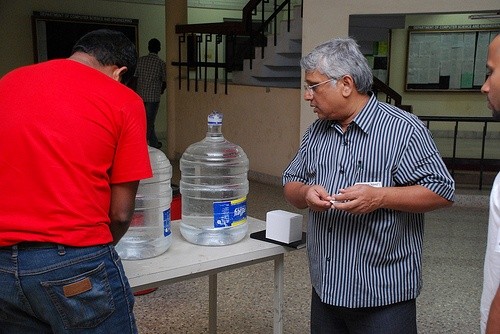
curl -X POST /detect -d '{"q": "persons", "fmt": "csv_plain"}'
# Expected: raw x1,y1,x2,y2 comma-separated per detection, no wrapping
477,32,500,334
0,29,154,334
132,38,166,148
282,38,455,334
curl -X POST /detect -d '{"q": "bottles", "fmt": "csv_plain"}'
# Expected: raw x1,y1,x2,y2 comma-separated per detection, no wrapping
180,113,249,246
115,145,173,260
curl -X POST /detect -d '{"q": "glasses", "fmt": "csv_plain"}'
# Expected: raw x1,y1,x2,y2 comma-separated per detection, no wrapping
304,78,333,93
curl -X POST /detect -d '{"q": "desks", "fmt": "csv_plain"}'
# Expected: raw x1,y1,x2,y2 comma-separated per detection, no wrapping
120,216,308,334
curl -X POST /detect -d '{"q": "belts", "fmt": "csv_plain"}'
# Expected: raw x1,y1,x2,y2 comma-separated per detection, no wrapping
8,241,57,250
